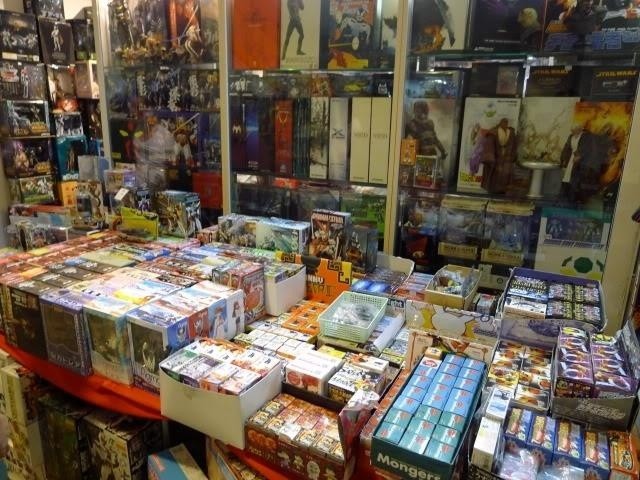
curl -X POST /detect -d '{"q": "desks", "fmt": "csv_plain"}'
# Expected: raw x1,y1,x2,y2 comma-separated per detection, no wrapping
1,335,384,479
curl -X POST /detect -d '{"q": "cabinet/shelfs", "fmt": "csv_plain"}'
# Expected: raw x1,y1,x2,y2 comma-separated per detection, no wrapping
90,0,225,229
222,1,403,254
390,1,640,337
